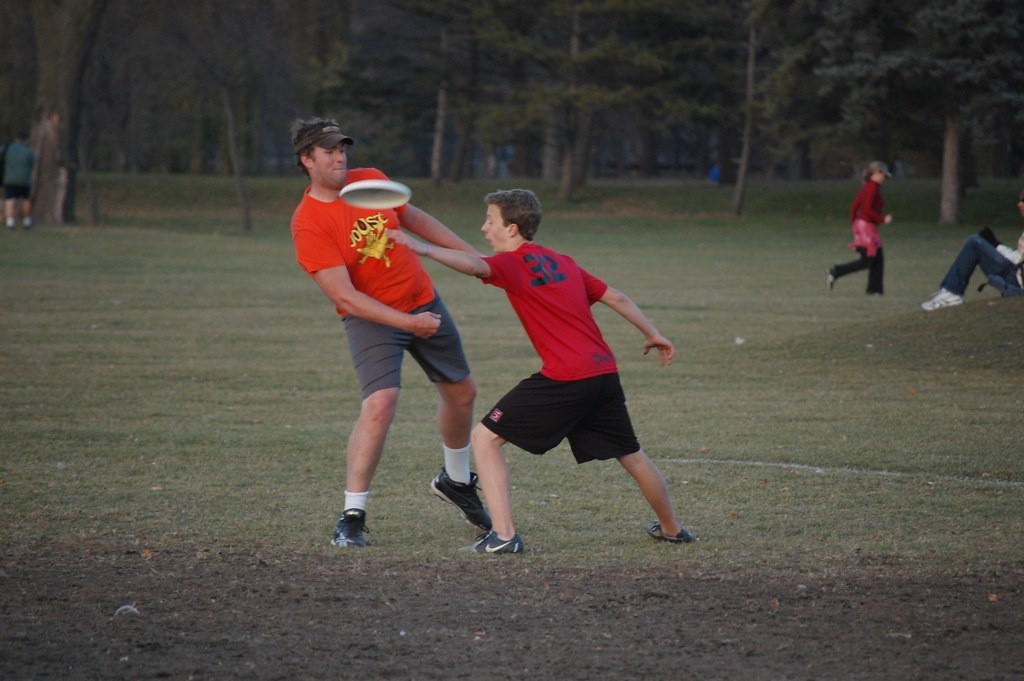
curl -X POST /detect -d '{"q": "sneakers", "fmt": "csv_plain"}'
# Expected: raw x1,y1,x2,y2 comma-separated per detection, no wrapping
329,508,370,548
645,520,695,545
431,465,492,531
921,288,964,311
457,528,523,555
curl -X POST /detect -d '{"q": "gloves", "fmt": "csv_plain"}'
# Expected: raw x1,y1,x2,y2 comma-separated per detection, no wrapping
980,226,1002,248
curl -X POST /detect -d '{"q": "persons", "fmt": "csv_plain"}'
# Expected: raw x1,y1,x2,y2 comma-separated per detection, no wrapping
921,190,1024,311
825,161,893,297
388,190,696,553
29,108,63,229
291,119,490,545
3,131,38,230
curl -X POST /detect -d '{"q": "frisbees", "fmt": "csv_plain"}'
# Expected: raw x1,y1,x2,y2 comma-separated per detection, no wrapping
338,179,413,211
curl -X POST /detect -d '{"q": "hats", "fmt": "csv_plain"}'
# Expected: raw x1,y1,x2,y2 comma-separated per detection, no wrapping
295,122,353,155
869,160,893,178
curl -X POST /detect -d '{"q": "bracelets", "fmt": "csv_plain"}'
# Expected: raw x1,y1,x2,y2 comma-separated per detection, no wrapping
425,244,432,257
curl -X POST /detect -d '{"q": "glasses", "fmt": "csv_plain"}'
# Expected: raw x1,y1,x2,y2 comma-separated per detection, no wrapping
1017,199,1024,203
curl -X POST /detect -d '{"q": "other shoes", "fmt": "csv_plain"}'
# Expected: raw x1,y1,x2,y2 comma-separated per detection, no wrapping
826,268,838,291
